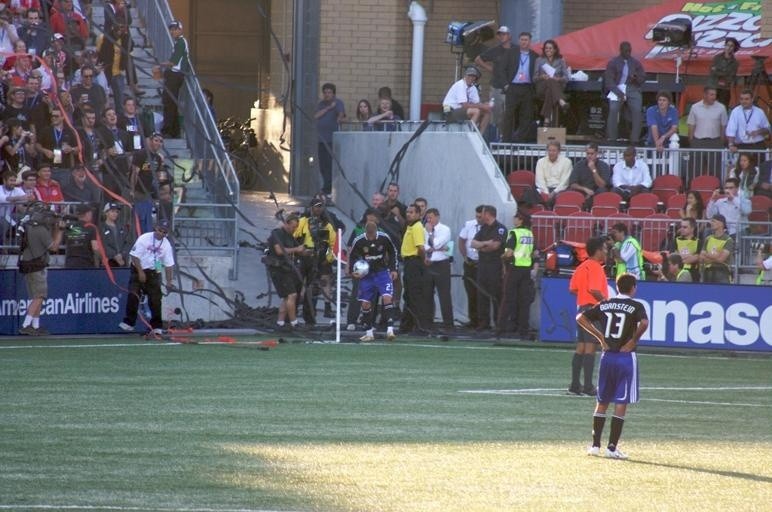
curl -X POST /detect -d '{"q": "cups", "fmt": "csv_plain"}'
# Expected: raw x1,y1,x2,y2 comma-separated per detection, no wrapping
81,94,88,103
152,65,161,80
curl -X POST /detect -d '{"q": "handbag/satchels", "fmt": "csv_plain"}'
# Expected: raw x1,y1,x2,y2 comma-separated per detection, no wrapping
16,257,50,274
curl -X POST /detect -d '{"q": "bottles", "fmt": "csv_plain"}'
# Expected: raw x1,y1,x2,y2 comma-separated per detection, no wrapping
445,241,454,257
489,98,495,107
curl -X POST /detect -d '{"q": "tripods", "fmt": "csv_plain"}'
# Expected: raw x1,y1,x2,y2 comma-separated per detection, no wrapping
748,64,772,111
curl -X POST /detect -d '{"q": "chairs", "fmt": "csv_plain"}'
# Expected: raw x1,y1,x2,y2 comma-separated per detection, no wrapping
507,170,772,259
1,2,185,255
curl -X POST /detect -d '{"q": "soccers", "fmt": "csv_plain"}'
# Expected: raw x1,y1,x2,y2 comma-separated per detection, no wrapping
353,262,370,275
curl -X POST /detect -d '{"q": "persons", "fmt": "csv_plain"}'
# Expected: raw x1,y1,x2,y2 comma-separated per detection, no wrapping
569,238,609,396
1,1,772,340
575,274,649,459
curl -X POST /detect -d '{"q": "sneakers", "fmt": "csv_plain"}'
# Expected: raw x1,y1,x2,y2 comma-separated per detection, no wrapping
119,322,164,335
276,312,397,331
35,326,50,336
360,335,376,342
18,326,37,336
584,386,597,395
400,327,537,341
588,447,601,456
386,332,396,342
605,447,628,460
568,386,582,395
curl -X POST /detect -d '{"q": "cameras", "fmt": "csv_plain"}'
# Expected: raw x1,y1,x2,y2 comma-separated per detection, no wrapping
643,262,669,273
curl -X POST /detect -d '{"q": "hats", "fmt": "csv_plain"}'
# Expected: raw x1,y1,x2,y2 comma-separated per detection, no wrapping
76,204,96,215
157,219,170,231
496,26,510,34
104,202,122,213
465,66,478,76
17,22,183,170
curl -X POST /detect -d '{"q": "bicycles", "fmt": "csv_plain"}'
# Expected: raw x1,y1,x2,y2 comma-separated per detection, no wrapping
218,115,264,191
